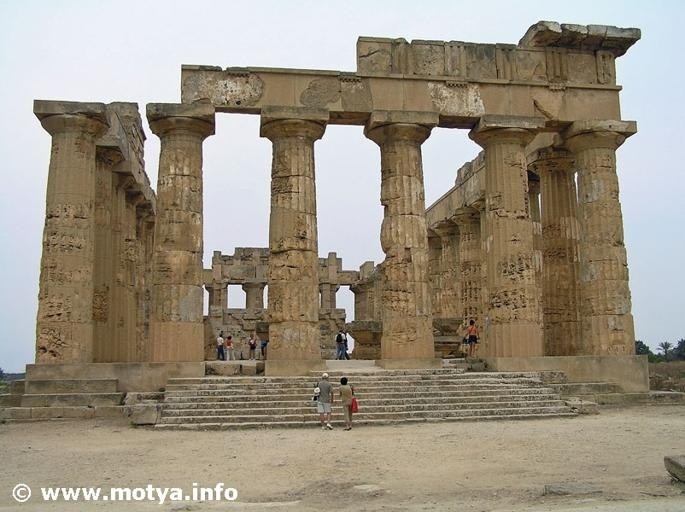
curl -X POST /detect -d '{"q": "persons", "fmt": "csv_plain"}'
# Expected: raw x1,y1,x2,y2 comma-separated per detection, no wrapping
339,377,360,430
339,332,350,360
248,334,257,361
335,330,346,360
225,335,235,361
217,334,225,360
467,320,478,358
317,373,334,430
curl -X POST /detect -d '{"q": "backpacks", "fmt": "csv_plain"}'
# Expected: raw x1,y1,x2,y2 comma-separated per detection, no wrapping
336,333,343,343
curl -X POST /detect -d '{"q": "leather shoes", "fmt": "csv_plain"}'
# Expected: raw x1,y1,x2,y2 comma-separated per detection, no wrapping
343,427,352,431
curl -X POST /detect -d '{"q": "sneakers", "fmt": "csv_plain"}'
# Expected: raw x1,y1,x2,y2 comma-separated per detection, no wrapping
321,426,327,430
327,423,333,430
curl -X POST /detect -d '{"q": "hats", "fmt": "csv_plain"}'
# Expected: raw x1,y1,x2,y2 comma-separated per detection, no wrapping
322,373,329,379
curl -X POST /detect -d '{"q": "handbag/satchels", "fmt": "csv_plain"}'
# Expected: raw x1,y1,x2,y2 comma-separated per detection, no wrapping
351,397,358,413
